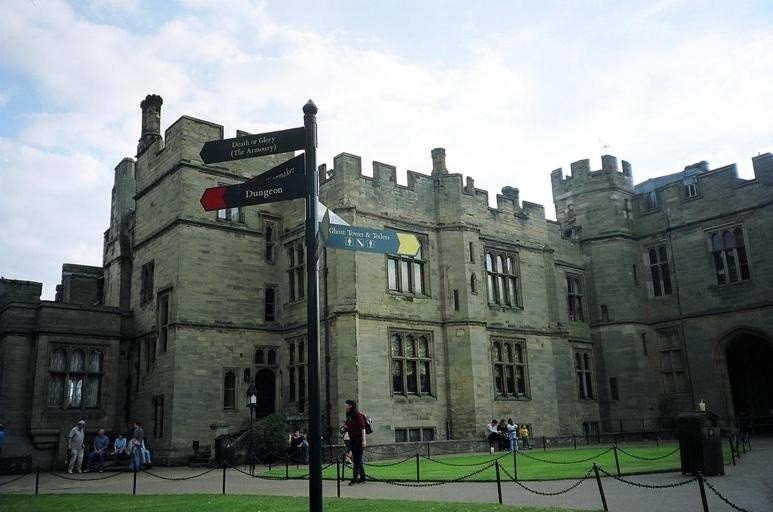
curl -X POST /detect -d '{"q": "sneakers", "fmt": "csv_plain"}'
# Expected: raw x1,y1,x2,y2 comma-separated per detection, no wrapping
347,480,366,486
67,469,140,474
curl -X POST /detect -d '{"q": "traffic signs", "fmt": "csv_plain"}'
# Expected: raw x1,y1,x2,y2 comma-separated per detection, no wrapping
199,126,422,257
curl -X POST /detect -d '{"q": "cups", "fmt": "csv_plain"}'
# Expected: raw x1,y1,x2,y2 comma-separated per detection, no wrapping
699,403,706,411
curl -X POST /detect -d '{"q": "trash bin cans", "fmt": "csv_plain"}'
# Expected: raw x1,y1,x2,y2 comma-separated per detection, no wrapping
673,409,723,477
214,434,236,469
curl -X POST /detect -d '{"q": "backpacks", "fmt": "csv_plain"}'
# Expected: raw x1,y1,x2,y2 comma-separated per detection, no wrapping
348,412,373,434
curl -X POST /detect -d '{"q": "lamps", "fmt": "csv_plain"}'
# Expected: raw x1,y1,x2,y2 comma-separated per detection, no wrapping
244,368,250,383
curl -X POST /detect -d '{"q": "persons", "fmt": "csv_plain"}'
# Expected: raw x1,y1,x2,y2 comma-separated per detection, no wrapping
497,418,510,451
484,419,504,452
287,429,309,464
112,432,127,465
519,424,532,450
140,439,151,464
125,421,143,473
65,420,86,475
506,418,518,451
343,399,366,486
83,427,109,474
0,424,6,448
342,431,352,464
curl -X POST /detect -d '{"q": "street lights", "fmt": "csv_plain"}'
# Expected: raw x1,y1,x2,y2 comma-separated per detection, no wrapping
246,383,257,473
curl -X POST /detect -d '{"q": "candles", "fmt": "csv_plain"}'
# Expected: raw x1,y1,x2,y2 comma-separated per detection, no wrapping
698,398,706,411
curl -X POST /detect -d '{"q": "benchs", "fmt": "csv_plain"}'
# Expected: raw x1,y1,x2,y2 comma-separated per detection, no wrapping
62,433,146,471
